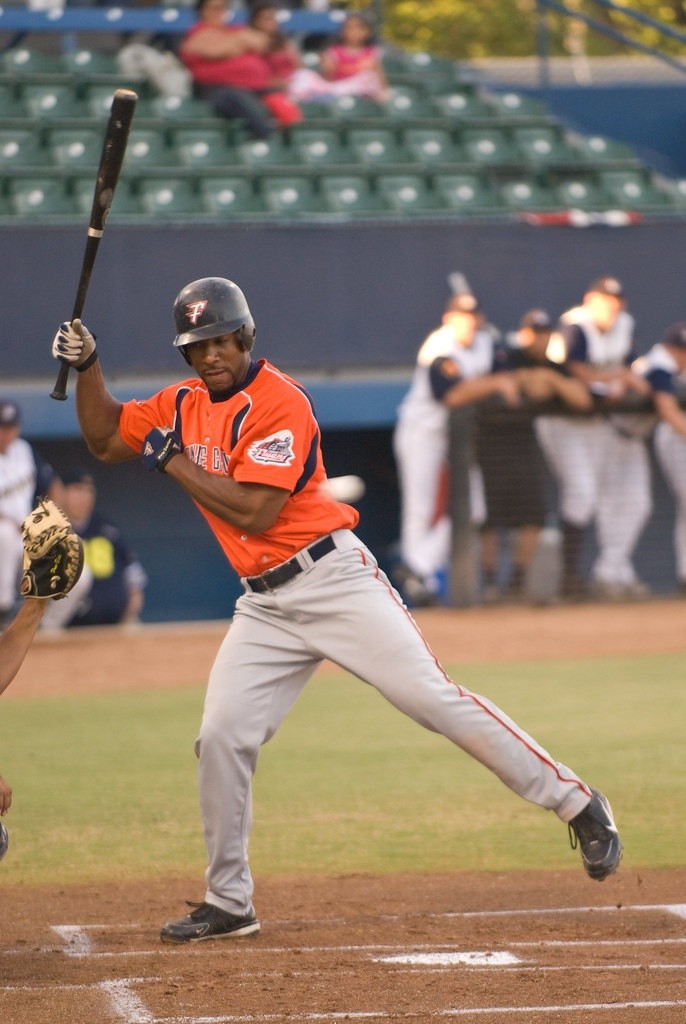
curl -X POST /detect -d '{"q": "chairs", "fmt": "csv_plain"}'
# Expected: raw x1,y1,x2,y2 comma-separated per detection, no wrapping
0,43,686,220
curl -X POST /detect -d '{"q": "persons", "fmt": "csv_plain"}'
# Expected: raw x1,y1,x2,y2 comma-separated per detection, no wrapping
1,1,686,816
57,279,622,942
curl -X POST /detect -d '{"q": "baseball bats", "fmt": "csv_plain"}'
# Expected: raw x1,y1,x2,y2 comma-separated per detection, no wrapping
48,85,141,406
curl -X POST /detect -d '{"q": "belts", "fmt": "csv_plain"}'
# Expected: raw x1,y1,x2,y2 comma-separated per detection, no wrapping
248,535,336,592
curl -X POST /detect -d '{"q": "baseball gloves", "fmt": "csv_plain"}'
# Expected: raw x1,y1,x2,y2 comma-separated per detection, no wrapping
14,488,88,608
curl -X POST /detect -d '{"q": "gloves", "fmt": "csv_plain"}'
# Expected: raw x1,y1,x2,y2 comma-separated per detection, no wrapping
140,426,183,473
50,318,97,372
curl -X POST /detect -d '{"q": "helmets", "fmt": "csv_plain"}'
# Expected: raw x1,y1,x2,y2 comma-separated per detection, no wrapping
171,276,257,351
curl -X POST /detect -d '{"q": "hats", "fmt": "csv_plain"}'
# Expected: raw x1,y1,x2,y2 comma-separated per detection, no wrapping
662,323,686,349
441,291,479,316
586,275,625,298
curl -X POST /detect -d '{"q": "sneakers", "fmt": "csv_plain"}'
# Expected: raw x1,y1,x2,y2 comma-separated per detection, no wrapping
566,789,623,880
160,900,260,945
